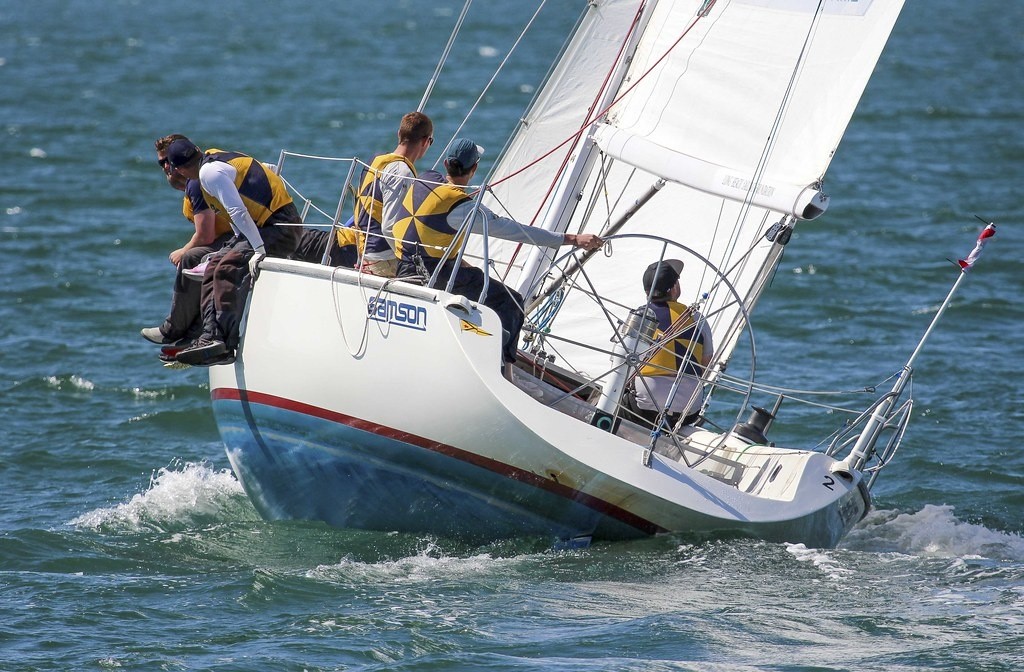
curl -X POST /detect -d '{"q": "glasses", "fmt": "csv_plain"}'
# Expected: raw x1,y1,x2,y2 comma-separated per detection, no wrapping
158,157,169,168
423,135,434,146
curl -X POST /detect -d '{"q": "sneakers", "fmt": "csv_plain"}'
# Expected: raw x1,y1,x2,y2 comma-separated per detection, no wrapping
176,338,226,364
191,349,236,368
182,257,211,281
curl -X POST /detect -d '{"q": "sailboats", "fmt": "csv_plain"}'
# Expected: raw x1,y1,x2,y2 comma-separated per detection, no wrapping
210,1,995,551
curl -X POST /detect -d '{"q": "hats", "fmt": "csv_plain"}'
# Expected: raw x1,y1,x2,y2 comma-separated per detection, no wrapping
168,139,198,175
447,138,485,168
643,259,684,298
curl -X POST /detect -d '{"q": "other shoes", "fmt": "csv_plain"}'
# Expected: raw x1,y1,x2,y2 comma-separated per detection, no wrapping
161,338,193,360
141,327,184,342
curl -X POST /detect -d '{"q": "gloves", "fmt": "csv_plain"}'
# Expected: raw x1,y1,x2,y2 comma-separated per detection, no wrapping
248,245,266,281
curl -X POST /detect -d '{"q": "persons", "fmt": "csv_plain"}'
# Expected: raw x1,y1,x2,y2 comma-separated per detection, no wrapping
392,137,603,381
182,214,355,282
618,260,712,430
354,111,435,277
140,133,304,369
